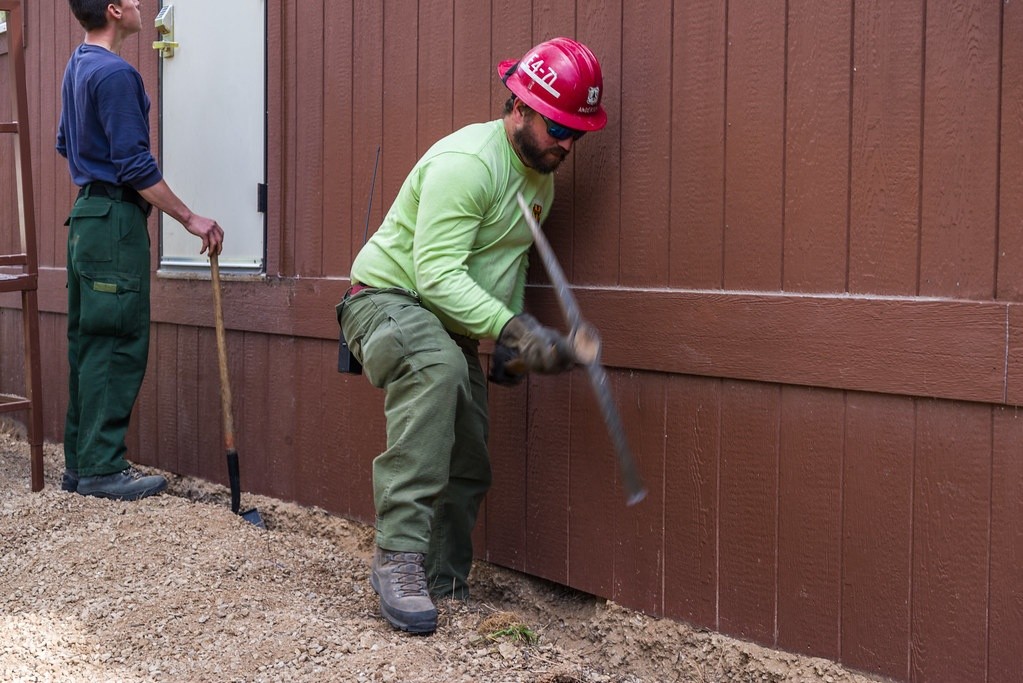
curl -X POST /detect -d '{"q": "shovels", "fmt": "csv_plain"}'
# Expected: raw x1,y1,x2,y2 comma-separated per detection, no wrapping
209,244,266,529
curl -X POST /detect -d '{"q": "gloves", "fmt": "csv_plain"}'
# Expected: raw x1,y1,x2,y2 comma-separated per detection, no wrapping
487,341,530,386
499,312,575,375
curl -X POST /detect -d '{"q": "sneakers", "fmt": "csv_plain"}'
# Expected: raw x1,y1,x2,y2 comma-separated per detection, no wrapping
62,467,78,492
371,545,438,632
76,466,168,501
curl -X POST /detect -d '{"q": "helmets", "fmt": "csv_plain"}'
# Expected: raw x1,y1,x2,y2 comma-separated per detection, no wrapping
497,36,607,131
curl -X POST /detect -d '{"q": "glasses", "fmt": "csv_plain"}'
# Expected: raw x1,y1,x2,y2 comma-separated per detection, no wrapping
540,114,588,141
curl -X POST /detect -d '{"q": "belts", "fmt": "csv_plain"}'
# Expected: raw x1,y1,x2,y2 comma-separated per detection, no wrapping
351,283,373,295
79,179,152,217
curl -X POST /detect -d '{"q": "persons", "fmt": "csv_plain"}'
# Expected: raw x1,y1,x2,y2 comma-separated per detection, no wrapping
340,38,609,638
55,0,226,497
